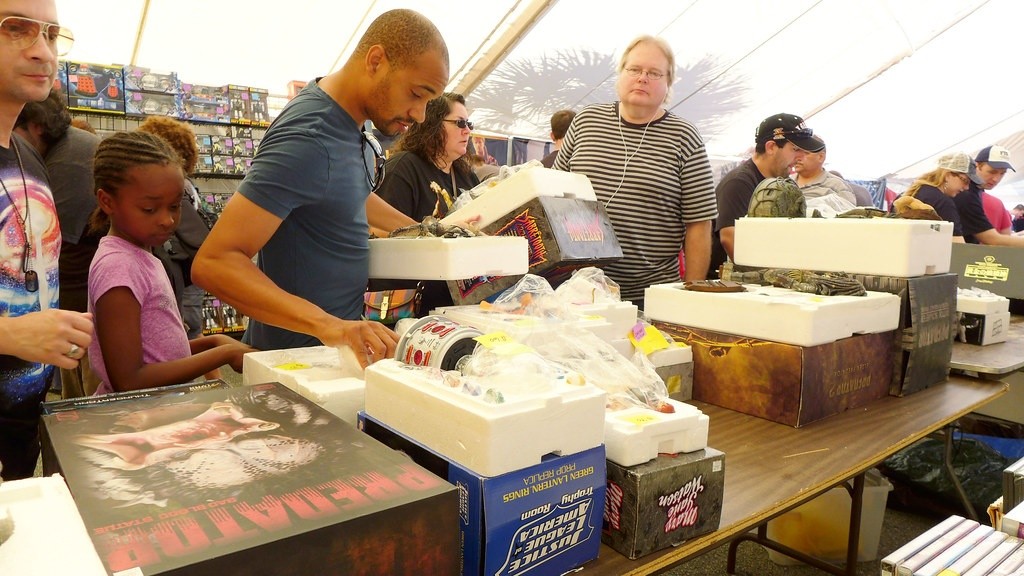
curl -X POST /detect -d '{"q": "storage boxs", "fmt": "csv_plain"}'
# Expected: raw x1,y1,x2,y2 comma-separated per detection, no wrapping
40,166,1024,576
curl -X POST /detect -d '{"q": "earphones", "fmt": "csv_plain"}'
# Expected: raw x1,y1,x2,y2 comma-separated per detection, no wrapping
666,86,669,93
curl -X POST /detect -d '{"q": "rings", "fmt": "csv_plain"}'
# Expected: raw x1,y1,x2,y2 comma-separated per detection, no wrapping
66,344,80,358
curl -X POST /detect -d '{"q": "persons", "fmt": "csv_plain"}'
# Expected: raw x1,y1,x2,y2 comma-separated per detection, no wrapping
890,152,985,243
0,1,96,483
951,142,1024,246
469,154,501,183
980,187,1012,244
189,10,450,371
87,129,261,396
1005,204,1024,232
11,86,112,313
828,170,873,207
549,36,719,311
790,134,857,207
371,90,482,316
701,113,825,281
138,113,222,380
540,110,576,168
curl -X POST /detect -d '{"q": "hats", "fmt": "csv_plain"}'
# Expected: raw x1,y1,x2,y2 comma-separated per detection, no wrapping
978,146,1016,173
755,113,825,155
939,152,985,185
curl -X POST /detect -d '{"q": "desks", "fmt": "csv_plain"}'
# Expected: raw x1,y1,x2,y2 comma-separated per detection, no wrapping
562,373,1008,575
924,311,1023,524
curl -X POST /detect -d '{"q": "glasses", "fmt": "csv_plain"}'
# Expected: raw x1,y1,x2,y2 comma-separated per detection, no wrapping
623,66,668,80
951,171,972,188
443,119,474,132
762,128,812,142
0,17,74,56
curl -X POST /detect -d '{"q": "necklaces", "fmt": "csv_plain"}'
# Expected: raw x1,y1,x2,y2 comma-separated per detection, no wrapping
0,133,40,292
